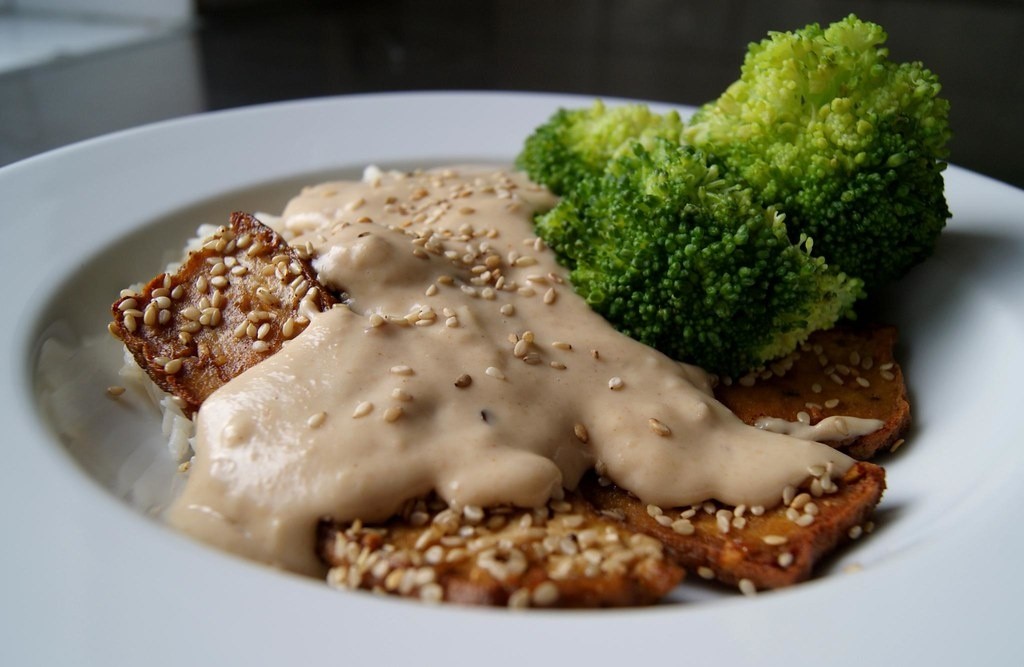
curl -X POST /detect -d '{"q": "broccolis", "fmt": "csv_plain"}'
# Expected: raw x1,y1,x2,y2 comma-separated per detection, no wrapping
508,13,956,379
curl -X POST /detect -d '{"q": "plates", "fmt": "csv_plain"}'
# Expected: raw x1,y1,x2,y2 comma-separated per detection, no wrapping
0,91,1024,667
